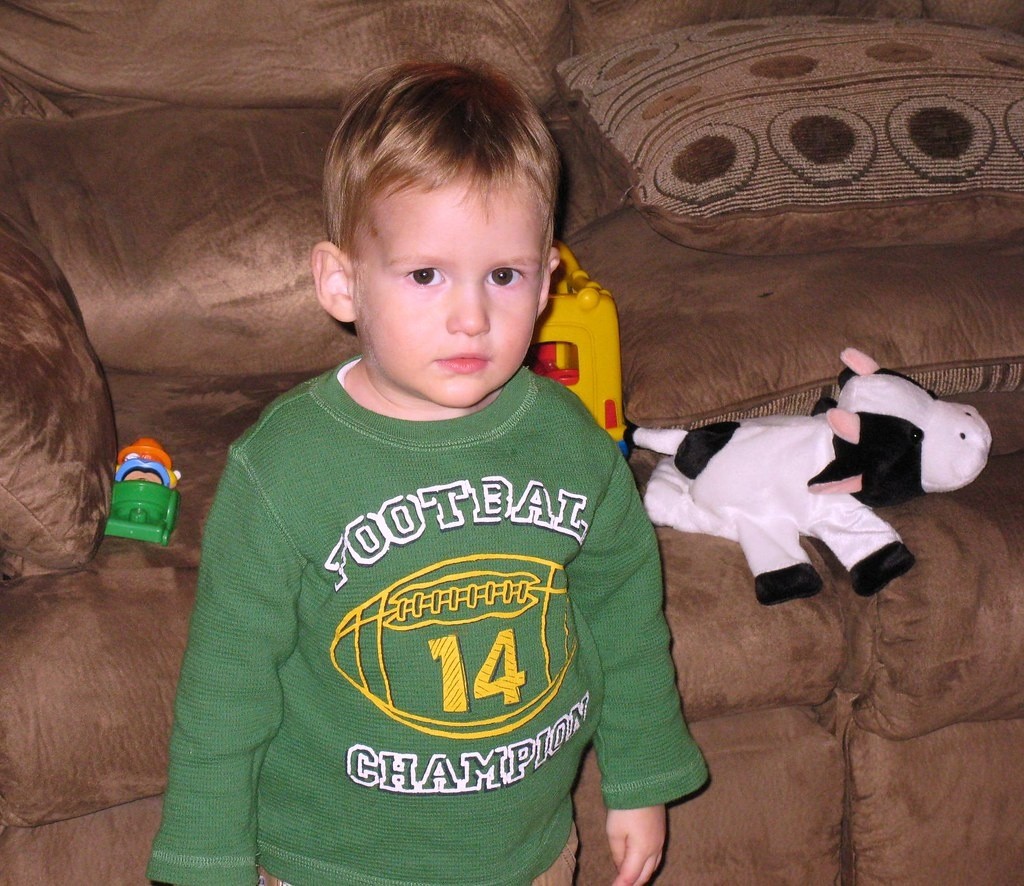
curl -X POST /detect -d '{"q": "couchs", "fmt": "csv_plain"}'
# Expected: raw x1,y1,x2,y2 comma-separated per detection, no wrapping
0,0,1024,886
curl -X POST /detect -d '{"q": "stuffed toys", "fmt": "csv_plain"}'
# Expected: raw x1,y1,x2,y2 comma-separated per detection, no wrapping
624,346,995,603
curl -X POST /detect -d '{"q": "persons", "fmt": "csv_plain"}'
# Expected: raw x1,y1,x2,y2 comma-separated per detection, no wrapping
142,60,710,886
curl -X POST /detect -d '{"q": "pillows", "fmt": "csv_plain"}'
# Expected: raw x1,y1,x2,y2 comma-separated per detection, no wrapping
551,20,1024,256
563,206,1024,431
